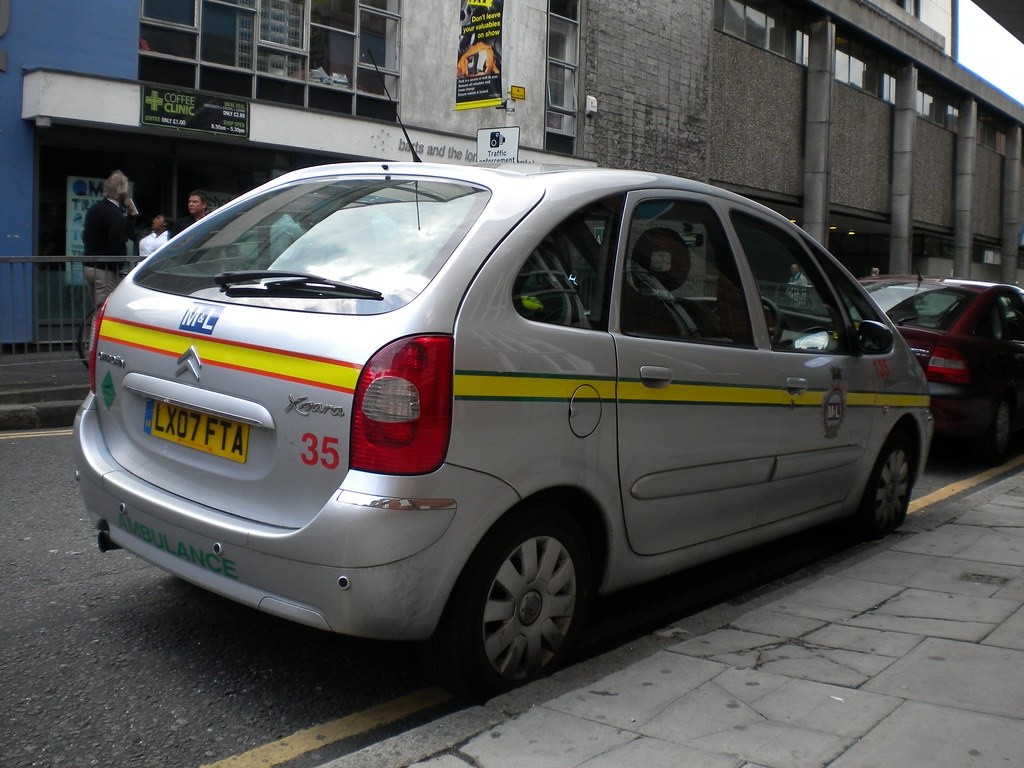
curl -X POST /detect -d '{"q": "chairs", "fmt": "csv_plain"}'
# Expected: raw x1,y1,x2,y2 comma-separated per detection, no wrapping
630,228,694,338
709,241,776,351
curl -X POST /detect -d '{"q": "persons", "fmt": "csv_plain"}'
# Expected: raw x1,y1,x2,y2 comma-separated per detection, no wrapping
139,214,172,262
82,171,141,314
170,190,208,238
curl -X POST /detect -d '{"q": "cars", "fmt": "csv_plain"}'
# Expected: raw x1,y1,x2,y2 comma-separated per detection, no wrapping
848,270,1023,467
72,156,939,709
